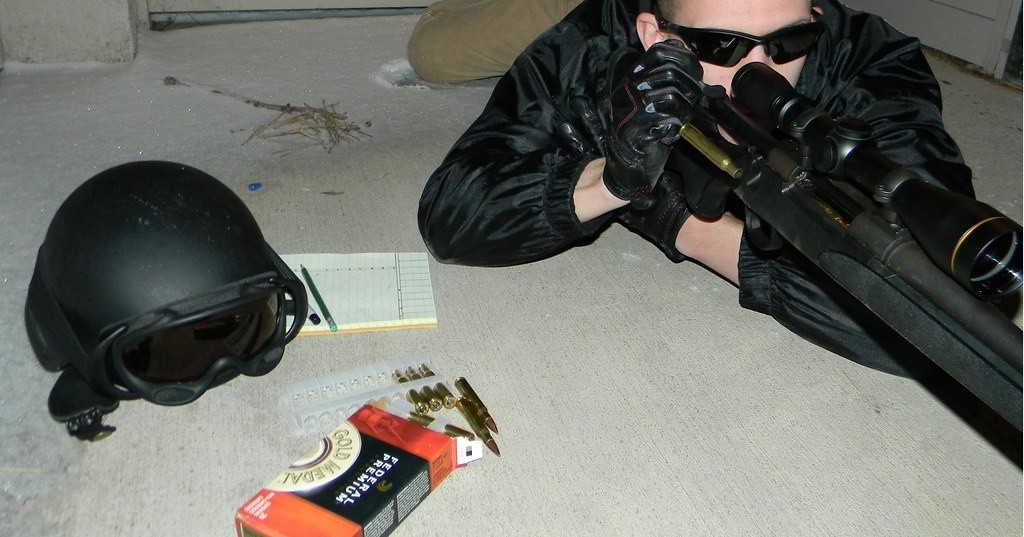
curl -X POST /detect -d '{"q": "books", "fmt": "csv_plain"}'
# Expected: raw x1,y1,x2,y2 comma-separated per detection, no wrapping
277,252,437,335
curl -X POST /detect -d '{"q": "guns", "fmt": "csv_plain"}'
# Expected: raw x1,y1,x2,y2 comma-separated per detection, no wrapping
600,41,1023,481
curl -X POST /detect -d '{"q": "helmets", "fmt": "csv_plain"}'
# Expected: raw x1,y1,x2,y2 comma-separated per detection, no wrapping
24,159,309,443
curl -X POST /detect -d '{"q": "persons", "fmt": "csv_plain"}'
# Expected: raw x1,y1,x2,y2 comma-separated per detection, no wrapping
417,0,977,378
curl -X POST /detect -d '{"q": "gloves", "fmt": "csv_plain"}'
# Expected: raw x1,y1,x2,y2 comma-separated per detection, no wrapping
556,61,695,263
599,37,701,202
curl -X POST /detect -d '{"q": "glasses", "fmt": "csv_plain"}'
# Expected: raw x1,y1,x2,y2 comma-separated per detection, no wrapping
110,287,286,406
644,1,823,69
